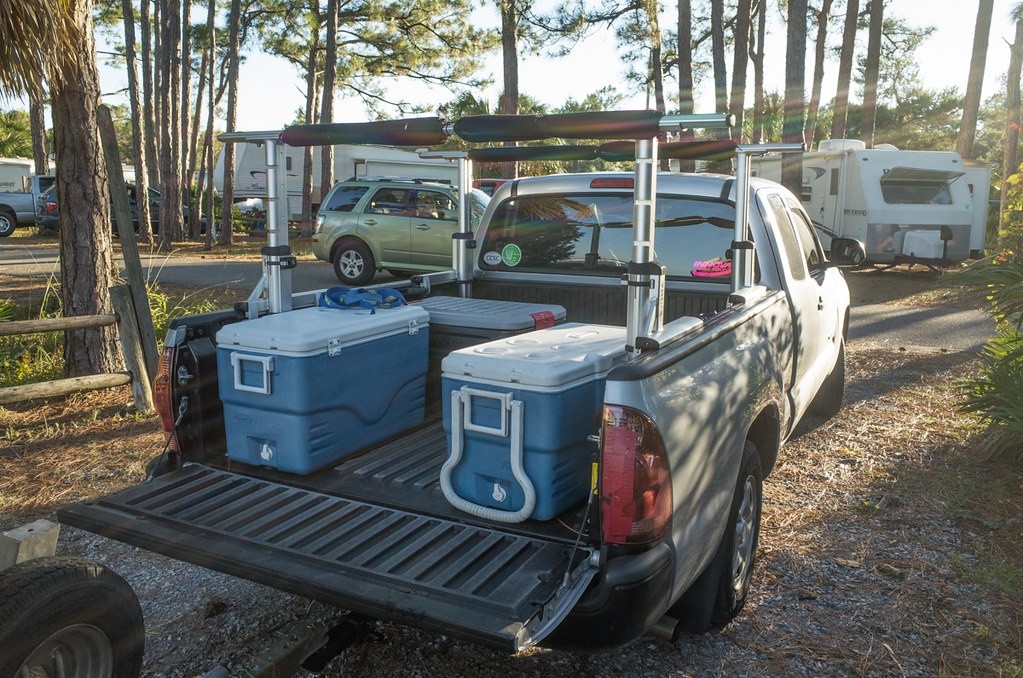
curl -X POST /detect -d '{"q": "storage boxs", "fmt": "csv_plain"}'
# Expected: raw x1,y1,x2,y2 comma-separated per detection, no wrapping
440,323,627,523
407,296,566,415
214,303,429,475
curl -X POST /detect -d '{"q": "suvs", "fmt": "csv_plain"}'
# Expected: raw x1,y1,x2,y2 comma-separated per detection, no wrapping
310,177,492,287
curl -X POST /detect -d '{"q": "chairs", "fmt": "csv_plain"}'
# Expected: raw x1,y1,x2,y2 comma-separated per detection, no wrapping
387,194,404,209
404,190,419,210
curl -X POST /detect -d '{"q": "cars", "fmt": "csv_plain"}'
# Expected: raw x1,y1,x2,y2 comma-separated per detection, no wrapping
473,178,514,199
35,177,218,237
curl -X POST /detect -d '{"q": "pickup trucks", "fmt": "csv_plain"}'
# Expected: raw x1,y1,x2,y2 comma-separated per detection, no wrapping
0,174,57,238
52,110,866,652
